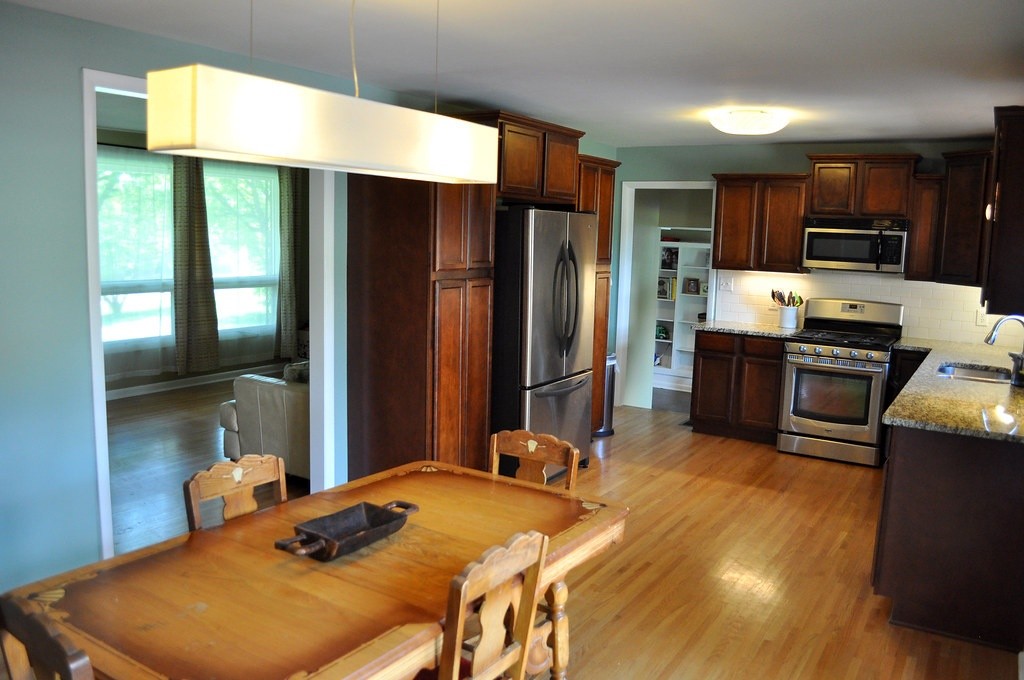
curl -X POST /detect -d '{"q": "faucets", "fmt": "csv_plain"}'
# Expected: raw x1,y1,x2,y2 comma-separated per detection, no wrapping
984,314,1024,386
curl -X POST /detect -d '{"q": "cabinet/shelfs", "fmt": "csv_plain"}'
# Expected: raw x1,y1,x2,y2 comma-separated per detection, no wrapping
690,331,782,441
652,240,711,391
347,112,622,491
712,105,1024,314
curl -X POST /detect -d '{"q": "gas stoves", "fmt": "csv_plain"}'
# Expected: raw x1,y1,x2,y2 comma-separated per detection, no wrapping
784,297,905,362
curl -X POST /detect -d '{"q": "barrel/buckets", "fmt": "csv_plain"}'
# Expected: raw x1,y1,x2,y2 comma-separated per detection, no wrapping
778,305,799,328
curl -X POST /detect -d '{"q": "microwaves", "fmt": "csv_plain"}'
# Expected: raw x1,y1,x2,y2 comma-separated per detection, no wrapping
802,215,908,274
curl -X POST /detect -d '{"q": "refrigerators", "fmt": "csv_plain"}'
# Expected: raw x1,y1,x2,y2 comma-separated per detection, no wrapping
491,206,599,479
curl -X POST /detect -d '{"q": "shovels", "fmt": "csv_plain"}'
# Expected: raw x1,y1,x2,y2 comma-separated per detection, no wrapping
795,296,803,307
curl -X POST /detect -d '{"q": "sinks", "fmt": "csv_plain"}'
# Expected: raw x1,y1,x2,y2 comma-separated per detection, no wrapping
934,361,1012,384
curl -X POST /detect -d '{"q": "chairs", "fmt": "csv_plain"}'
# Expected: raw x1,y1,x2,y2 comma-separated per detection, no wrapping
0,591,95,680
183,454,287,530
488,430,580,492
438,531,570,680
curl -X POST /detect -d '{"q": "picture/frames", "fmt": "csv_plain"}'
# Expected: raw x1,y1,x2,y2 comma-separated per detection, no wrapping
700,282,708,295
686,278,700,294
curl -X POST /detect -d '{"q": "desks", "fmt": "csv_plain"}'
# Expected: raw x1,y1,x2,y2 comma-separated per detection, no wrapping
0,461,629,680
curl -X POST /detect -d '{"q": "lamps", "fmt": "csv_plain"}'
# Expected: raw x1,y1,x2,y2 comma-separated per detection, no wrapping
708,108,789,136
145,0,498,184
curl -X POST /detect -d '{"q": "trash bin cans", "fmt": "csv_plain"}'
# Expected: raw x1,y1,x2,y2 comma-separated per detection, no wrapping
593,353,617,435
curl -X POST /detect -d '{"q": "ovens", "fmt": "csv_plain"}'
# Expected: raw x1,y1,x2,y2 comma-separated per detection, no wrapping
776,352,891,468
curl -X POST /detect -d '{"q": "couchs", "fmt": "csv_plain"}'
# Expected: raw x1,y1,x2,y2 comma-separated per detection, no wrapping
220,361,310,479
